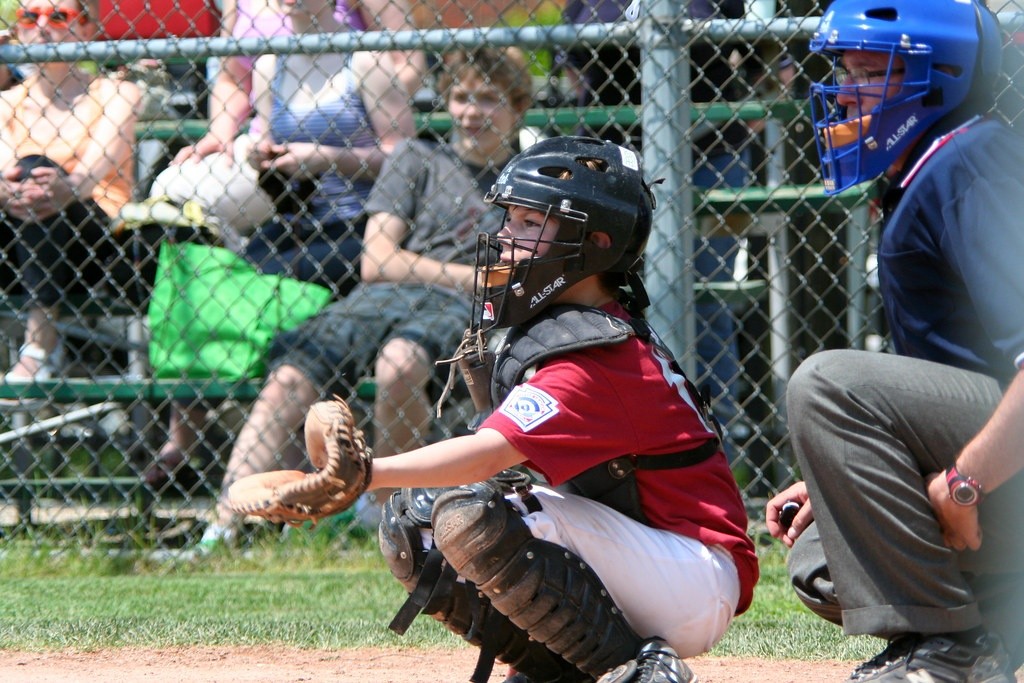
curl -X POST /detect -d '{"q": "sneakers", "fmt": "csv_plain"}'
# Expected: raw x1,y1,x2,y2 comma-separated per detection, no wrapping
503,662,596,683
839,629,1012,682
634,635,697,683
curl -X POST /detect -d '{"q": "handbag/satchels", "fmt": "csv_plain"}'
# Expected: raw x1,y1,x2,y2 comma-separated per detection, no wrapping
144,242,335,382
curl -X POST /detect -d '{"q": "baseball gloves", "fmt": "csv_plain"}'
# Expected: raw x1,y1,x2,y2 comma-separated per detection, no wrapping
214,398,375,526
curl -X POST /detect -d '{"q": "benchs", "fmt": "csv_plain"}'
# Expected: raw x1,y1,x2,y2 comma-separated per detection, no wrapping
0,374,468,407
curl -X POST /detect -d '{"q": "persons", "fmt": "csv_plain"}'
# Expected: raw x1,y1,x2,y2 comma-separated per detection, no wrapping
0,0,417,483
150,0,427,247
228,136,760,683
201,46,531,551
766,0,1024,683
558,0,794,459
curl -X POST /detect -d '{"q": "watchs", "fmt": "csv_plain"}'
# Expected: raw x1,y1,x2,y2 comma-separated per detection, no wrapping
946,463,985,507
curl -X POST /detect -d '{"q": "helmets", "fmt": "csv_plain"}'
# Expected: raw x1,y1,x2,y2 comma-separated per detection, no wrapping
469,135,665,338
810,0,1003,193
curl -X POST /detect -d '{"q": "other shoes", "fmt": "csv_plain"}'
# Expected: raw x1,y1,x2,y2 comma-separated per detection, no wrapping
0,339,68,412
284,482,387,543
182,524,243,561
147,448,193,481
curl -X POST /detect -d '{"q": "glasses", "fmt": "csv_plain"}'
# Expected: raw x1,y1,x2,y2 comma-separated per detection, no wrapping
15,8,89,28
834,65,941,89
